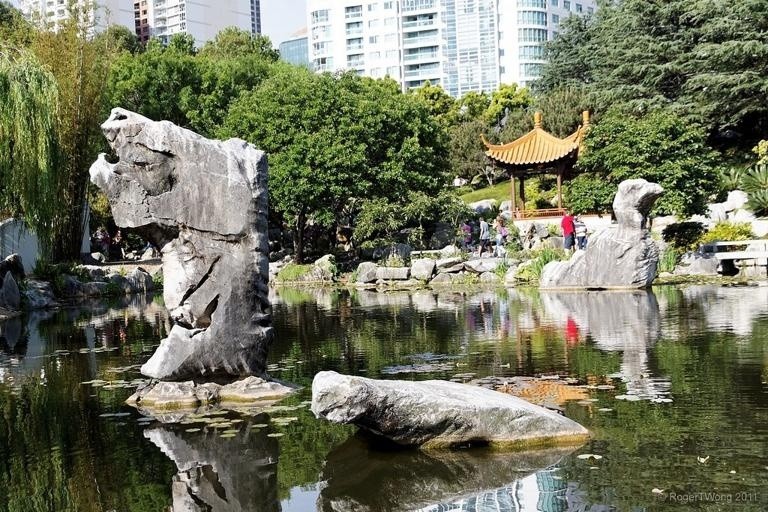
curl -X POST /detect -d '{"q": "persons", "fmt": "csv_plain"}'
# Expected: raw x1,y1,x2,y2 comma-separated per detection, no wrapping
561,212,576,258
477,216,494,259
573,216,587,250
460,220,473,253
492,216,506,258
